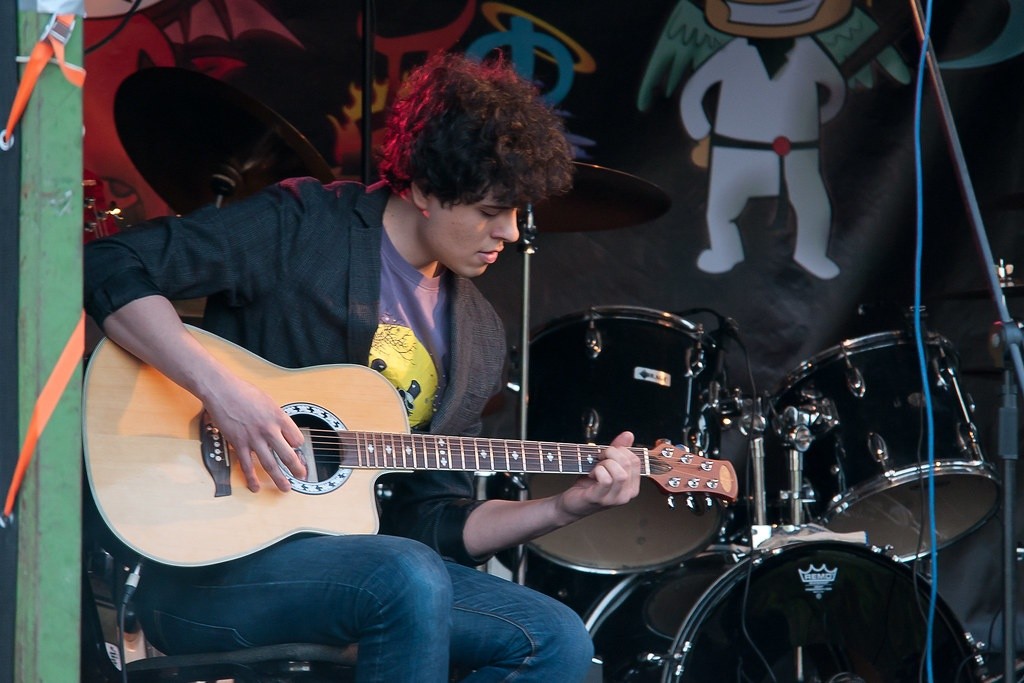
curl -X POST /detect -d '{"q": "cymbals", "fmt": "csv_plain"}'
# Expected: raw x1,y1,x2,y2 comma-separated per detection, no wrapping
945,284,1023,299
113,67,338,208
516,160,671,232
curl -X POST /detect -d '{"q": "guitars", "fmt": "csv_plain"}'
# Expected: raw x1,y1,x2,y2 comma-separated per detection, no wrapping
82,319,738,567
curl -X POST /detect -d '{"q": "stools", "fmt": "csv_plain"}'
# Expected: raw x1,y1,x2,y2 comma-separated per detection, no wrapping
125,642,359,683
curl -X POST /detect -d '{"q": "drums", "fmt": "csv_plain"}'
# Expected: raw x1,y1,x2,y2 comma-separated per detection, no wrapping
497,303,739,575
760,327,1001,561
578,530,979,683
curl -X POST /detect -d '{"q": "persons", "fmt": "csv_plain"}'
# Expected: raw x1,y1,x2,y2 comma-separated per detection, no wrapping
84,53,641,683
